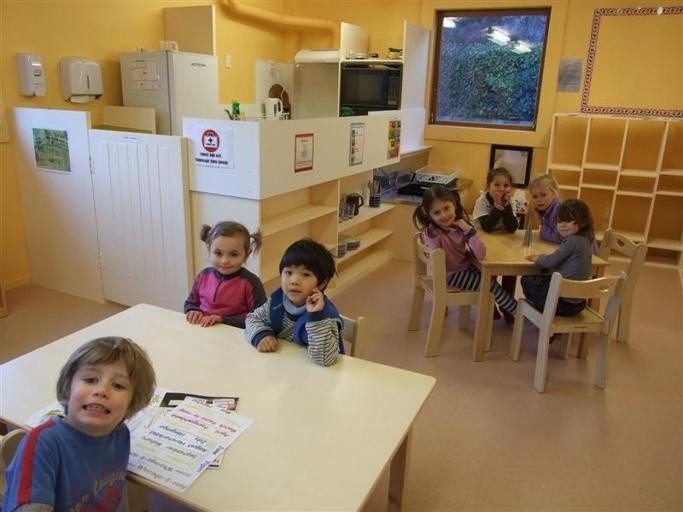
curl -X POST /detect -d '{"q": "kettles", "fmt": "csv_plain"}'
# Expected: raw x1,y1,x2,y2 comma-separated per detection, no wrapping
264,97,284,121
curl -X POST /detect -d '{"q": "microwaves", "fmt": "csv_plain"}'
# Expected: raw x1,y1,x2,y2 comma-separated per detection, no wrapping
341,61,400,110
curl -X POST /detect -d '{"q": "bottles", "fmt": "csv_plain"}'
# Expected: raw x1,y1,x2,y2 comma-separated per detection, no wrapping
231,99,242,120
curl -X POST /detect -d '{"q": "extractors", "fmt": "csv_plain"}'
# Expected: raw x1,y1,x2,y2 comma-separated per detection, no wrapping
293,47,340,65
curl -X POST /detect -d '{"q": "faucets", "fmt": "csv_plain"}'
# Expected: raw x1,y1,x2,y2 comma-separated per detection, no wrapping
224,107,235,119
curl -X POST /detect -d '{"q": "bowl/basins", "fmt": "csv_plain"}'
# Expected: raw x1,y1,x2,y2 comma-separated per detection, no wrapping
338,237,361,258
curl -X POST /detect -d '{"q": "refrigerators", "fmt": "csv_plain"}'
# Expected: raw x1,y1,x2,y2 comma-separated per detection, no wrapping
120,51,218,134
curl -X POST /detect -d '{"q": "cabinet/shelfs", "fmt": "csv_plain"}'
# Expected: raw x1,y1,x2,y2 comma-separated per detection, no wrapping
367,178,474,266
187,167,391,315
543,108,682,271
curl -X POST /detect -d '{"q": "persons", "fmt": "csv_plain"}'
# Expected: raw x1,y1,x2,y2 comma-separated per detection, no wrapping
2,335,157,512
183,220,268,329
520,199,594,345
412,183,529,323
528,174,599,279
244,237,345,367
471,167,520,321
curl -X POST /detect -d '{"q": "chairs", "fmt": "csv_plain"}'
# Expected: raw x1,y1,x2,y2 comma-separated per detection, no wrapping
0,425,28,512
335,310,370,361
581,227,649,343
505,270,628,393
405,231,495,359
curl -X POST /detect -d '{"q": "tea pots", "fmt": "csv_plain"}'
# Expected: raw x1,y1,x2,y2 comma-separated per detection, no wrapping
346,191,363,217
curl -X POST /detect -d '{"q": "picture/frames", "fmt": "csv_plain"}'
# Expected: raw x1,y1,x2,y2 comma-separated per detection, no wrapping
484,142,534,190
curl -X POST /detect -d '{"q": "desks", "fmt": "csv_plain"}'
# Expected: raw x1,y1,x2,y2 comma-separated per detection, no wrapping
458,225,611,364
0,301,440,512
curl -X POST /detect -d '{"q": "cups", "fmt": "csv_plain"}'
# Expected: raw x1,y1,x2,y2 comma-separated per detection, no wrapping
338,203,356,220
369,193,381,208
